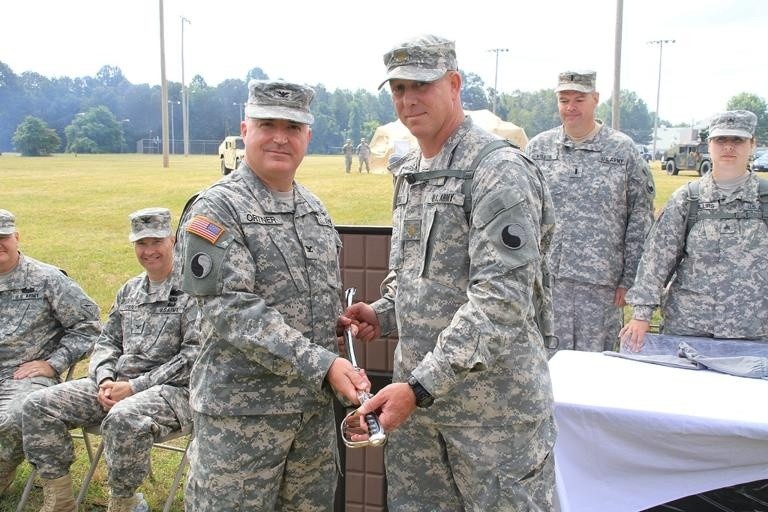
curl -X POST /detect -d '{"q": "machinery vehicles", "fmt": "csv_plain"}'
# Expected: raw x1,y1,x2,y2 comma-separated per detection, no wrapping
218,136,247,176
662,140,713,180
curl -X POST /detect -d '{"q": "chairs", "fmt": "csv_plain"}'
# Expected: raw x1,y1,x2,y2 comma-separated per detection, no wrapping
71,423,197,512
15,359,99,512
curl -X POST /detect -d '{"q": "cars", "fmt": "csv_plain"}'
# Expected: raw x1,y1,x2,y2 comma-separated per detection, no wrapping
636,145,650,162
749,147,767,172
647,148,664,160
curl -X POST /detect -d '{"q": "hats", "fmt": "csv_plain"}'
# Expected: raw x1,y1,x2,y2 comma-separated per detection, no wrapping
245,78,317,126
553,70,598,95
376,32,458,95
127,206,174,243
705,108,758,141
0,209,19,235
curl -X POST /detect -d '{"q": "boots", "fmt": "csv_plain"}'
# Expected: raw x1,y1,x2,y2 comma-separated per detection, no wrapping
106,490,137,512
38,474,77,512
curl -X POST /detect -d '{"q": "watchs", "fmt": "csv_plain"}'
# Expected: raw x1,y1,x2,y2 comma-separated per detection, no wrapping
407,375,433,407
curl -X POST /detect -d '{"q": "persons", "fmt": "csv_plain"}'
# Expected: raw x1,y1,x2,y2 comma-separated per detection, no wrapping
342,36,562,512
23,208,204,512
356,136,372,174
617,110,768,350
342,138,353,175
526,69,656,352
1,204,101,503
182,86,372,511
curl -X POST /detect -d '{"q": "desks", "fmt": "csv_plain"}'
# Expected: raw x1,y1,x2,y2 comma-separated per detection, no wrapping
545,346,766,511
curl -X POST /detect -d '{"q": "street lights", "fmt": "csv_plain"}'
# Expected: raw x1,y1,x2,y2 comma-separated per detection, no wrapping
486,48,512,115
232,101,247,132
649,39,677,161
121,118,130,152
181,16,192,157
168,100,181,155
